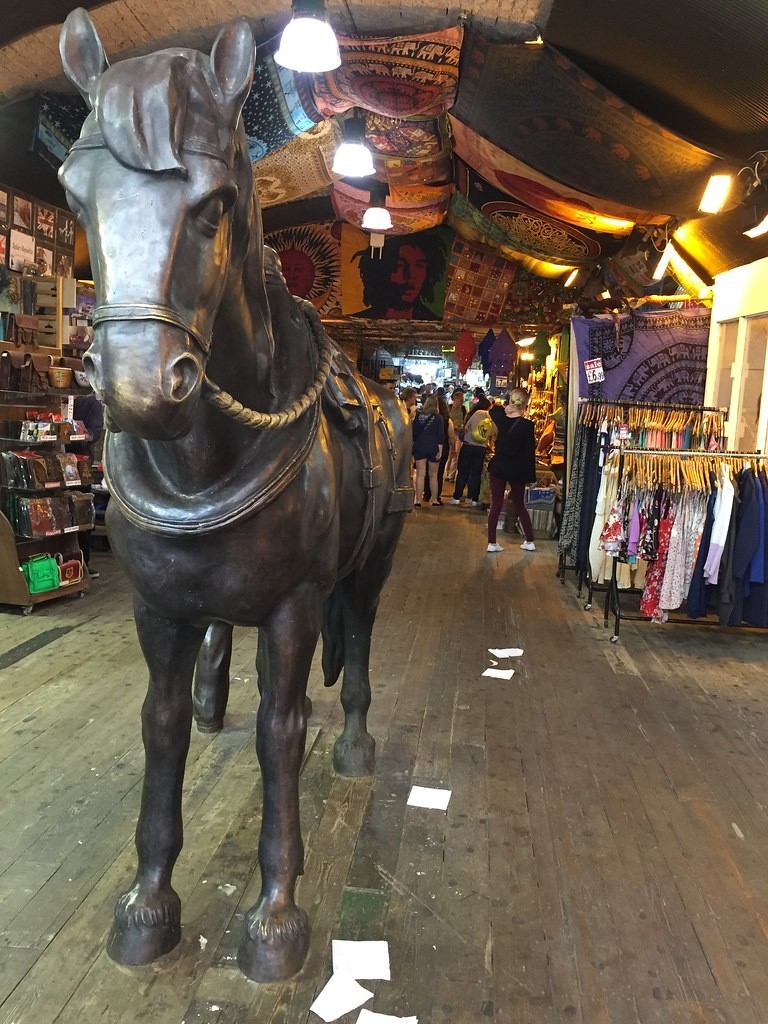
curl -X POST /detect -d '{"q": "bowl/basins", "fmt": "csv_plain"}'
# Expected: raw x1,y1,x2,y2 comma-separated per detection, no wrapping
74,370,90,388
49,366,72,389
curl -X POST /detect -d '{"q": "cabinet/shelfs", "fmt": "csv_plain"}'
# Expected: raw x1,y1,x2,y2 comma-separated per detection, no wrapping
0,391,95,613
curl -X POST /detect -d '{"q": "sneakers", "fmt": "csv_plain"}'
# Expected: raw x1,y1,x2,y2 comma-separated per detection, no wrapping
472,501,480,507
465,498,472,504
447,497,460,505
519,540,536,550
487,542,503,552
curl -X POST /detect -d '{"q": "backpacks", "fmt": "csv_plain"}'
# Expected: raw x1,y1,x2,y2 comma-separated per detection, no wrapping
470,411,493,443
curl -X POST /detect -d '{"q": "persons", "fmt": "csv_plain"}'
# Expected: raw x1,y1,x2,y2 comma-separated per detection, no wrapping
65,396,103,578
486,388,537,551
378,372,498,509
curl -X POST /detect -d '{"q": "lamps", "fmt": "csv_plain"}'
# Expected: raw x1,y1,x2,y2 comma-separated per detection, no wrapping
561,269,579,287
515,332,536,346
273,0,342,73
646,238,674,280
360,188,393,231
331,116,376,178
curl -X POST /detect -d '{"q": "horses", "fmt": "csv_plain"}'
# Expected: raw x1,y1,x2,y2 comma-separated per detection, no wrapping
54,6,412,987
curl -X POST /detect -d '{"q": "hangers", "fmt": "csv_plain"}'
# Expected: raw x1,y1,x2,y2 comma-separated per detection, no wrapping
578,397,768,496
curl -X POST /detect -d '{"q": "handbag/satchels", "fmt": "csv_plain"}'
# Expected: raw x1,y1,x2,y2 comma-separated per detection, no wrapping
20,549,84,594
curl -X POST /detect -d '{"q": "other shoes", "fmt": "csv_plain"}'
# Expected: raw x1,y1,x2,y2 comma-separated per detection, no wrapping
88,568,99,577
423,497,429,502
415,503,421,508
445,478,449,481
432,503,443,507
449,478,454,483
